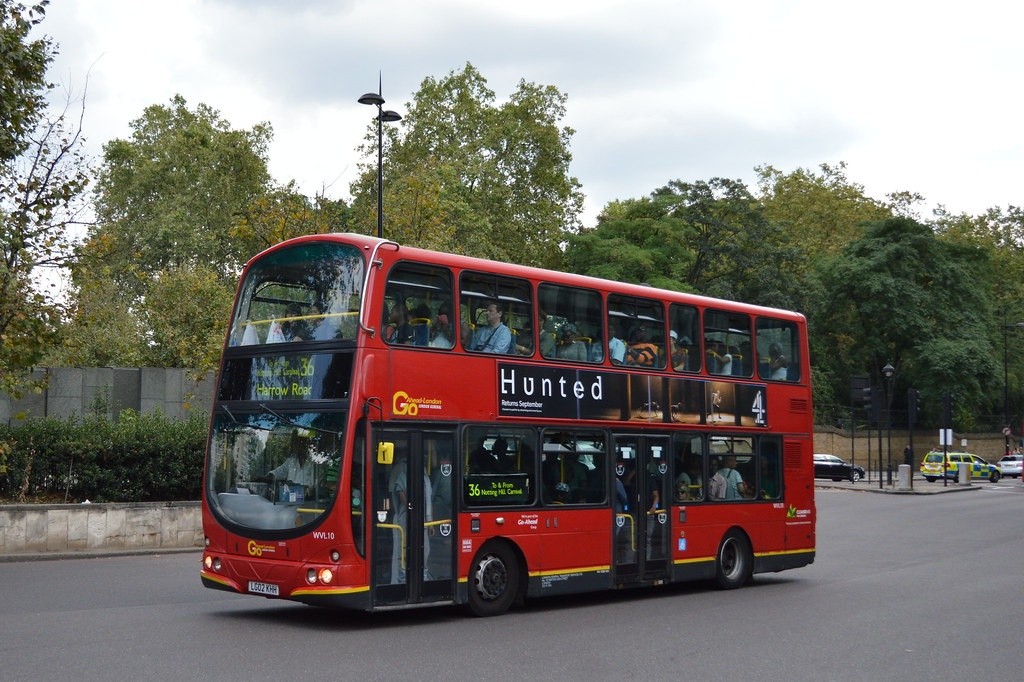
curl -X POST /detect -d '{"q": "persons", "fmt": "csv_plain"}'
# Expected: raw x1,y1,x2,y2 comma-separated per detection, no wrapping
592,322,625,364
382,300,468,346
542,452,603,503
388,446,452,584
625,321,658,367
659,329,686,370
264,434,341,495
706,340,732,374
904,442,910,464
469,436,515,474
761,454,776,498
282,302,336,341
557,323,588,361
471,302,512,353
516,309,554,356
616,460,659,563
1009,447,1024,455
675,455,746,501
768,342,788,380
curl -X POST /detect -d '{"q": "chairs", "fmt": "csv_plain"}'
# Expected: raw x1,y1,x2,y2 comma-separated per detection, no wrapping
382,315,798,384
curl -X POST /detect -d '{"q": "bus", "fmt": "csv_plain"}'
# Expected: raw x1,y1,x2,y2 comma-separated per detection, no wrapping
200,232,816,617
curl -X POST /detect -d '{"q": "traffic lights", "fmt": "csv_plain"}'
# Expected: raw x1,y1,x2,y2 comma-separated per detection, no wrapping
948,392,954,416
911,388,920,414
862,384,874,412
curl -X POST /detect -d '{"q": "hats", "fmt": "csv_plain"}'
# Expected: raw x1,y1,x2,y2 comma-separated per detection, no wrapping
669,330,678,340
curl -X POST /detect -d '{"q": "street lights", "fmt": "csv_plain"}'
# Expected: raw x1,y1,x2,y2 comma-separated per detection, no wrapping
881,359,896,484
358,93,402,238
1004,322,1024,455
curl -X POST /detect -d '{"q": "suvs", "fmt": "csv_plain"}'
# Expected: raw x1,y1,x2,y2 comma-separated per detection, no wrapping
920,447,1003,484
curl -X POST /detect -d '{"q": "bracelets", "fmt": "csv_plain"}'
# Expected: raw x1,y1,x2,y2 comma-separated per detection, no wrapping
521,346,524,352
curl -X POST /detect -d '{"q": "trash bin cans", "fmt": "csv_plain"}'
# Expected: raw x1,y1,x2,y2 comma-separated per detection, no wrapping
958,463,971,486
898,464,911,491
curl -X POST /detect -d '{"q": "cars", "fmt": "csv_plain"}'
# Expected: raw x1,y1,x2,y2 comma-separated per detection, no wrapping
814,454,866,482
997,454,1024,478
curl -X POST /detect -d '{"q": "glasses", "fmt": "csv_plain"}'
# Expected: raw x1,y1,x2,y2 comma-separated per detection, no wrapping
676,491,684,495
562,333,573,339
